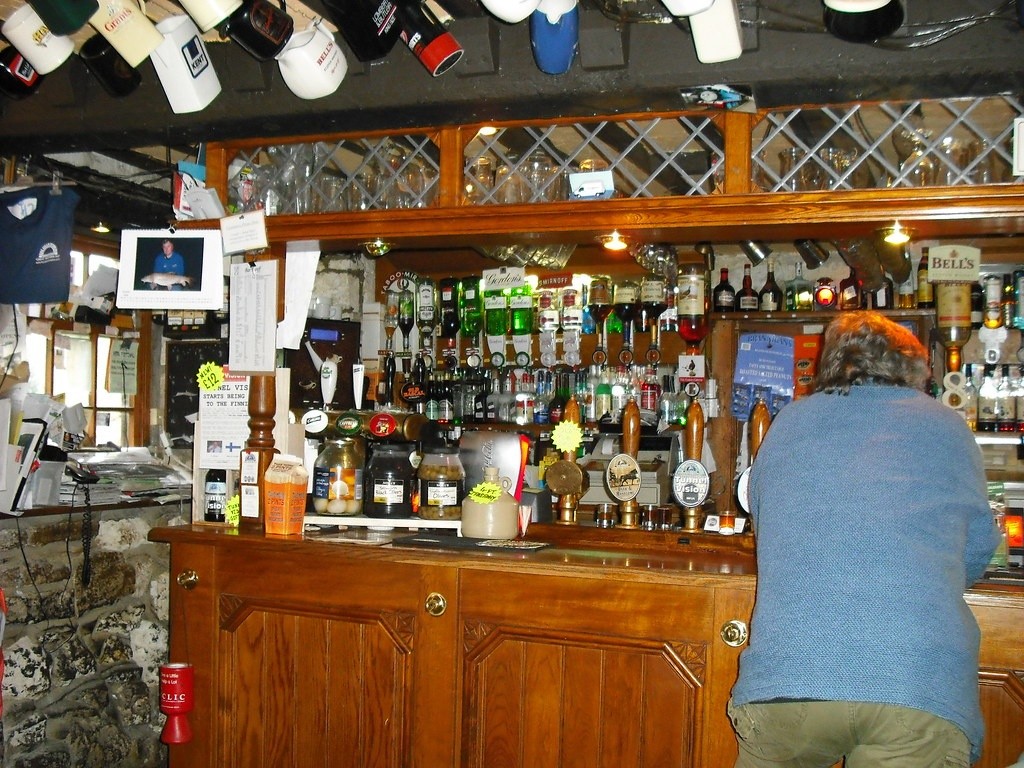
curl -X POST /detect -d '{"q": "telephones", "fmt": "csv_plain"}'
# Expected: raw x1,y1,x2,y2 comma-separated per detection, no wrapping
64,457,99,485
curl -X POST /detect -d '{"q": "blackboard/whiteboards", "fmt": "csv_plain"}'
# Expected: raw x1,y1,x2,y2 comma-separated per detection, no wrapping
163,340,230,448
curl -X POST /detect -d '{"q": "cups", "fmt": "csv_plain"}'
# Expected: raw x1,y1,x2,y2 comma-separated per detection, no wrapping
719,511,736,535
245,121,1024,216
596,503,615,528
640,504,673,531
0,0,576,116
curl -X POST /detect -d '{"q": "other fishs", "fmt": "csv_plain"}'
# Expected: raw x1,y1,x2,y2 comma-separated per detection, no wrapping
142,273,192,291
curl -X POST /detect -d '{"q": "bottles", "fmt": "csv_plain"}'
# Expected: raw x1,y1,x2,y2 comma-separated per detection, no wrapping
812,276,838,311
596,0,904,63
713,267,735,312
899,260,914,309
204,468,228,522
917,247,934,309
734,264,758,312
871,263,894,309
311,437,522,541
840,269,860,309
923,270,1024,436
785,262,812,311
758,262,784,312
377,261,713,428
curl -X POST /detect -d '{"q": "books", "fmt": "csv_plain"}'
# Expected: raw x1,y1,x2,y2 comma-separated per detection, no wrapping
0,392,194,515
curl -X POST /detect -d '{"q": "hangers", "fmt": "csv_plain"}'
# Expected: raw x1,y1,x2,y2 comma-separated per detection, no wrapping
0,152,78,195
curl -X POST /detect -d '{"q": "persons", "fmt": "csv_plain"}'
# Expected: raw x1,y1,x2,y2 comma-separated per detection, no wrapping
723,317,1001,768
151,239,185,292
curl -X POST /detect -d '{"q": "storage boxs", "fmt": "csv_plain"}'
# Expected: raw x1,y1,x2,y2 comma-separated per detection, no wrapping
30,461,66,509
263,478,307,536
579,459,670,507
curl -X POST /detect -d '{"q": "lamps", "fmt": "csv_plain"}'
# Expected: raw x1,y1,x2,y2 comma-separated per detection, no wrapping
357,242,396,257
874,226,920,244
593,234,630,250
89,220,112,232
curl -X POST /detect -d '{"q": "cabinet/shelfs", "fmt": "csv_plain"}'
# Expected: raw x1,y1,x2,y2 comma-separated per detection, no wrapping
162,89,1024,767
